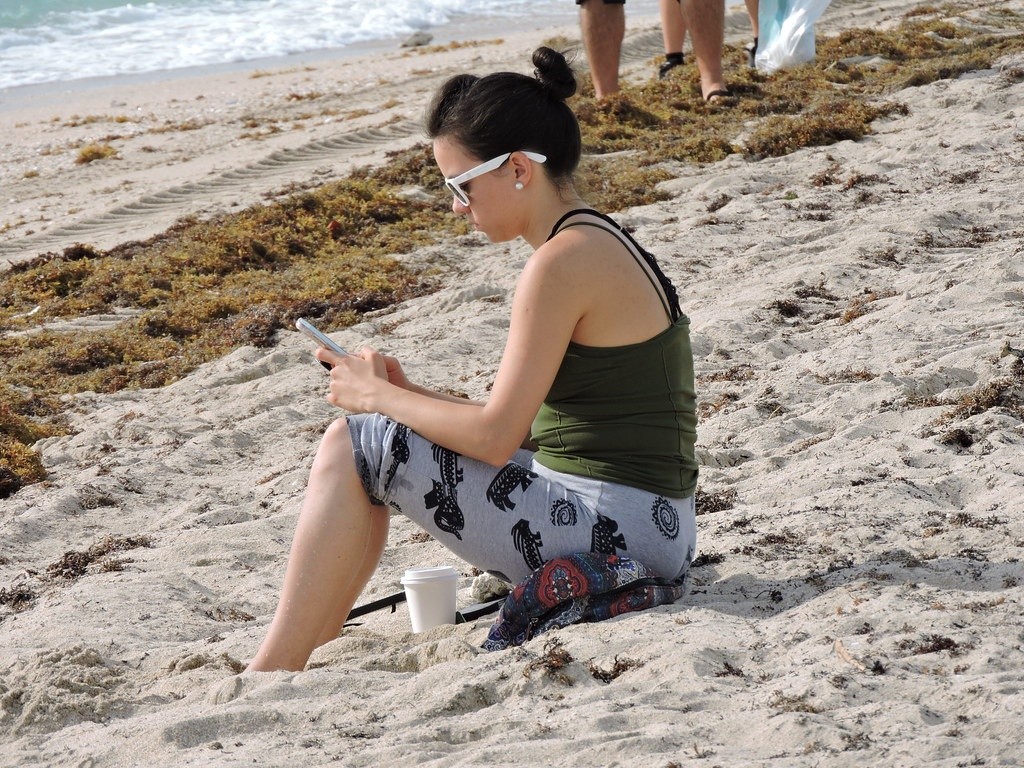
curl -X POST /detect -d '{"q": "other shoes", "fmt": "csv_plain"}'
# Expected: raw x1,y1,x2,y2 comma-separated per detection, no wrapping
659,61,685,80
749,47,762,68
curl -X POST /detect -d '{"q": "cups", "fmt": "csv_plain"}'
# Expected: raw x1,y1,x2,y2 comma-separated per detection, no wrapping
402,566,457,633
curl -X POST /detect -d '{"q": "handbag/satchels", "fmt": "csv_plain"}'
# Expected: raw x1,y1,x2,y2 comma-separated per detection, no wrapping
482,552,685,652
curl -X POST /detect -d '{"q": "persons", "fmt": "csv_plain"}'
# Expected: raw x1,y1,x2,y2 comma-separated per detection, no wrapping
238,45,700,672
573,0,829,105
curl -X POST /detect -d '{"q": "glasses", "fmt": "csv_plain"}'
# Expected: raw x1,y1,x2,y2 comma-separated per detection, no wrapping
444,151,546,207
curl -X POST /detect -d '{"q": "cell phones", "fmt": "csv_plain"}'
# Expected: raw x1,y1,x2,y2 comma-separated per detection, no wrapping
296,318,347,354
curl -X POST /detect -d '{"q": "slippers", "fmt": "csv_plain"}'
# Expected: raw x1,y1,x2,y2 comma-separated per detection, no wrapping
706,86,734,107
